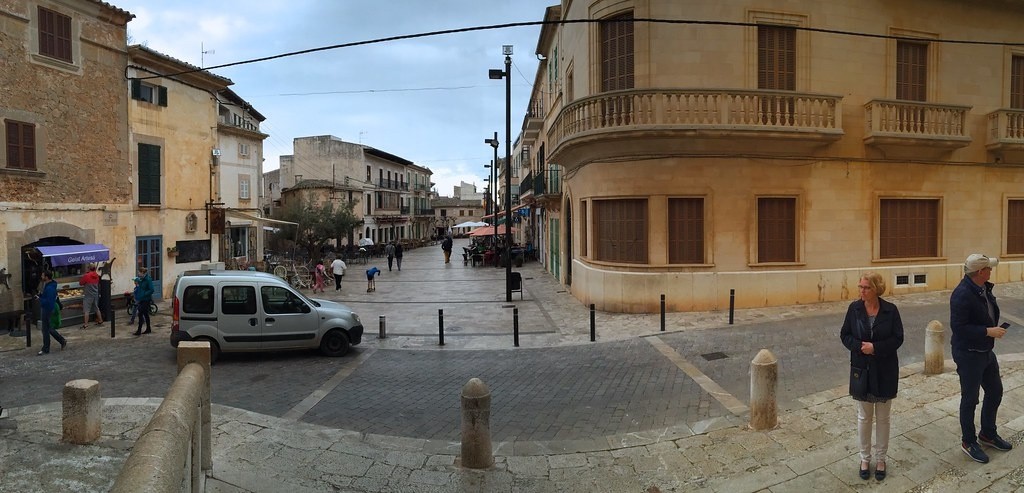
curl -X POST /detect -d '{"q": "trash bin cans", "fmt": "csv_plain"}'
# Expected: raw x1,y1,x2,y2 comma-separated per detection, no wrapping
509,272,521,289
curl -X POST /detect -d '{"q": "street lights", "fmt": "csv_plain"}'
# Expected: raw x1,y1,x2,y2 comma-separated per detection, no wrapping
489,68,512,302
484,138,499,268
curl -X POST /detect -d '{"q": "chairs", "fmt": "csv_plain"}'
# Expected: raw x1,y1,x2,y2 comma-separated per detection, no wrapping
273,235,432,290
463,238,531,269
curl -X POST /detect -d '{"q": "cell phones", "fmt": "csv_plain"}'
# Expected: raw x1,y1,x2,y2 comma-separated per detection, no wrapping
1000,322,1010,329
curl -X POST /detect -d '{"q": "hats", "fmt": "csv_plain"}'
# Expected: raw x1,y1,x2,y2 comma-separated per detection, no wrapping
964,254,999,273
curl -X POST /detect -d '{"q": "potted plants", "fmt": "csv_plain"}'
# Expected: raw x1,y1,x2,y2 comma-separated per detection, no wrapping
167,246,180,257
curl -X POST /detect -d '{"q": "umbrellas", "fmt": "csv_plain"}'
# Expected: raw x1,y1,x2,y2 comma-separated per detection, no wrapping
464,224,516,236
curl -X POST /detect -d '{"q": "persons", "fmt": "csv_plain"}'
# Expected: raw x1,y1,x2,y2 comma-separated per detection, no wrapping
128,267,154,335
841,271,904,481
312,260,326,294
330,254,347,292
949,253,1013,463
385,242,394,271
366,267,380,291
441,235,452,263
79,264,104,329
33,270,67,355
395,243,402,271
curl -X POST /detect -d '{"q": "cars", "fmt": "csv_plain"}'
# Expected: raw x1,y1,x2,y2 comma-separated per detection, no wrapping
171,276,364,357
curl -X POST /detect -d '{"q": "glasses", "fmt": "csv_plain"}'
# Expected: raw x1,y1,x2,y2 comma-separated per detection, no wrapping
858,285,871,290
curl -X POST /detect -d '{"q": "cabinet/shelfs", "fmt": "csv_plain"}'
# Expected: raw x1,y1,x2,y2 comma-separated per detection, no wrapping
59,296,99,328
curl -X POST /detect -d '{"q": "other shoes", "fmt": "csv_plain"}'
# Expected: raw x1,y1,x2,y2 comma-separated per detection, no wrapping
389,268,392,272
336,287,343,292
127,320,134,325
80,324,89,330
133,331,141,336
398,268,401,271
444,258,450,263
874,460,886,482
39,350,45,355
321,290,325,293
94,321,104,327
859,460,870,480
367,288,373,292
142,330,152,334
61,339,68,351
312,289,317,294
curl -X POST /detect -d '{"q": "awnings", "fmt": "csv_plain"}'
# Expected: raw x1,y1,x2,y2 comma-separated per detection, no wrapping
481,203,528,219
35,244,110,267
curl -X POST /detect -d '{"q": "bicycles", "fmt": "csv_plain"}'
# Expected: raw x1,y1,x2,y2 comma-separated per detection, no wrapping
127,299,158,316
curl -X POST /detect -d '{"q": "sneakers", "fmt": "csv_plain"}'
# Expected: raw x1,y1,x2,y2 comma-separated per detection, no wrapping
961,441,989,464
978,428,1012,451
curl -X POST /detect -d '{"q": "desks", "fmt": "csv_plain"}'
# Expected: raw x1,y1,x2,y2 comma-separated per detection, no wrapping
270,261,281,268
